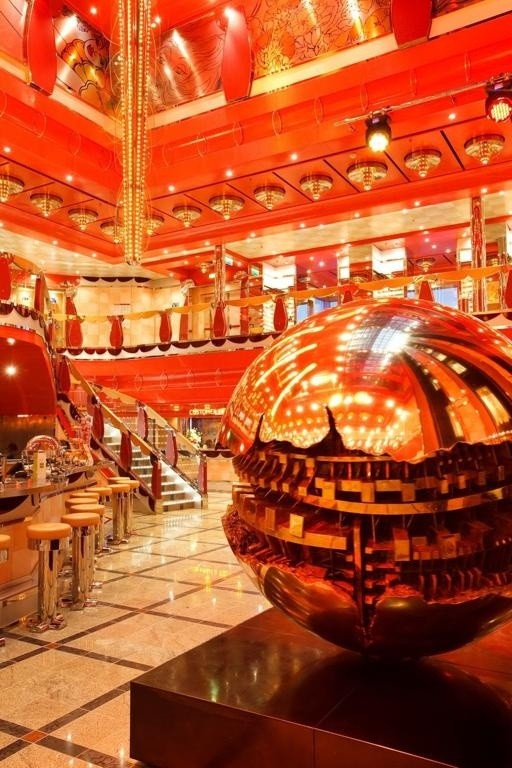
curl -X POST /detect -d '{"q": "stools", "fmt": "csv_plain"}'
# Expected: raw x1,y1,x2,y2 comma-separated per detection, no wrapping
0,476,143,647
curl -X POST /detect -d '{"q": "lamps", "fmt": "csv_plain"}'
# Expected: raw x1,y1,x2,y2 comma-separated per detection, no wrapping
486,76,512,126
345,160,389,191
171,199,203,228
463,135,505,165
365,115,392,154
299,173,331,201
209,183,246,221
404,148,442,178
0,172,164,246
253,174,286,211
